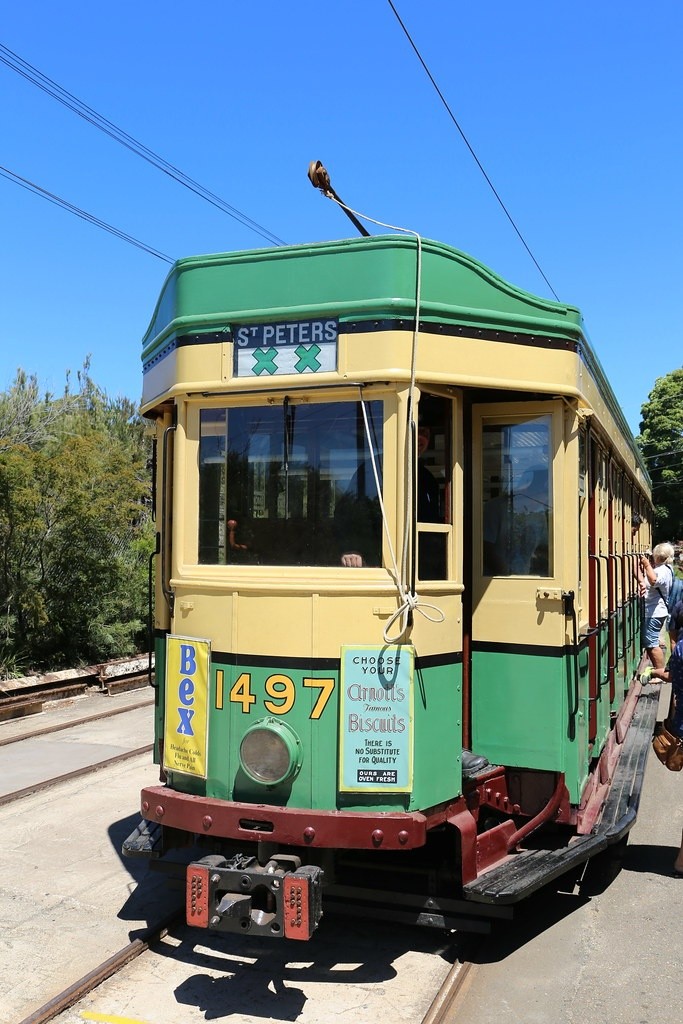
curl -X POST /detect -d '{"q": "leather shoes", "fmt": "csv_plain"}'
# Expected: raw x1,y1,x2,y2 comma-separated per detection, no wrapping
462,748,489,773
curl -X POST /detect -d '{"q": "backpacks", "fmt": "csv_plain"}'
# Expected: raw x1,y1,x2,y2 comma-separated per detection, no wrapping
668,601,683,643
656,564,683,614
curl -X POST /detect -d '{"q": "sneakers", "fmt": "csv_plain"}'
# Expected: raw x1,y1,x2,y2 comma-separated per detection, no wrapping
639,666,656,686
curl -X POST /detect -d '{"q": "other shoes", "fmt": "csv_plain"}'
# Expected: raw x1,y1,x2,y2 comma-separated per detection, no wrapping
648,677,667,684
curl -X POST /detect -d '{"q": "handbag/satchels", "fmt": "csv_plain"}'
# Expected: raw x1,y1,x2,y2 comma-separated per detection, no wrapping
653,718,683,771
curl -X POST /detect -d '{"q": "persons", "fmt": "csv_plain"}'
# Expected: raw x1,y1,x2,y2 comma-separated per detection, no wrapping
483,466,550,574
664,640,683,873
333,413,440,568
640,543,683,686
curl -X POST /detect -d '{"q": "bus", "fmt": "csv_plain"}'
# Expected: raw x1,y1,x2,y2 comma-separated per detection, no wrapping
121,160,662,941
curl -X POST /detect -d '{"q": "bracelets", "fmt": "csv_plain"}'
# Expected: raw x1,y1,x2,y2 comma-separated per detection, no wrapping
645,565,648,569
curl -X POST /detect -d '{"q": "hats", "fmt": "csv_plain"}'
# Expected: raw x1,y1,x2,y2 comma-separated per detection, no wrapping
418,415,430,440
